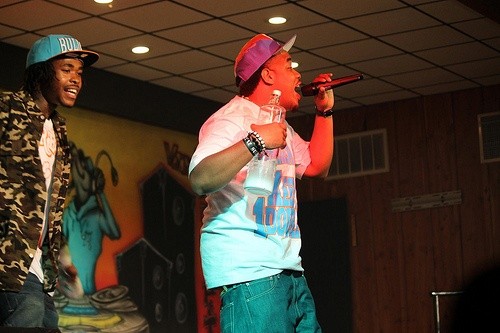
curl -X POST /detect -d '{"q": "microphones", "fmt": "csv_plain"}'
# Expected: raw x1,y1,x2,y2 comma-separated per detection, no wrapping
301,73,364,97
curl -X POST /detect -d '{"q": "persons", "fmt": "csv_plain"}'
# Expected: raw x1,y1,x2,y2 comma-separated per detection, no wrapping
0,34,99,333
189,32,334,333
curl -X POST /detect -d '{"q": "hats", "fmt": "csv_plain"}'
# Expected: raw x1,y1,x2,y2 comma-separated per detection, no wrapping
234,33,298,85
26,34,99,68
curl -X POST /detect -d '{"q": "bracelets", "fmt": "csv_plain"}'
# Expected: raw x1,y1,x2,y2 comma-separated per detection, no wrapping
247,130,265,152
243,137,258,156
249,134,263,152
315,105,334,116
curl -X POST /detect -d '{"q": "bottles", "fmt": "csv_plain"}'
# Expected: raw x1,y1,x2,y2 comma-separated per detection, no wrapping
243,88,286,195
